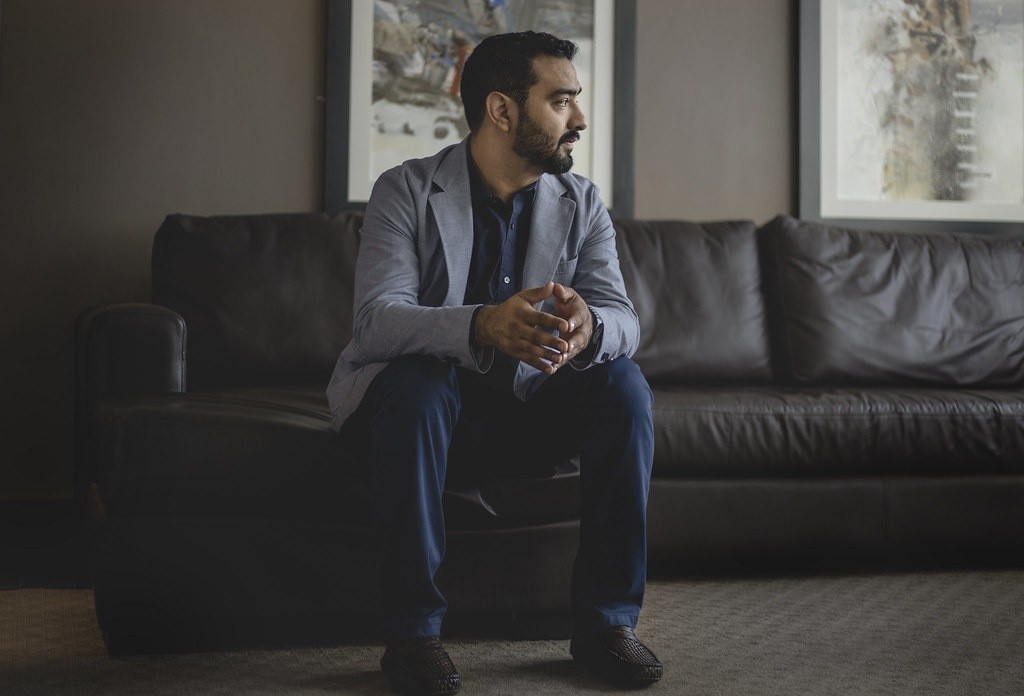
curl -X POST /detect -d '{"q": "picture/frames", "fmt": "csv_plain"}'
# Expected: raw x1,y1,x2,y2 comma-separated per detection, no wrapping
320,0,638,217
798,1,1024,222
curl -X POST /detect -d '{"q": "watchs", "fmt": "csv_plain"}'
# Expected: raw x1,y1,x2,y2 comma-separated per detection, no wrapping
585,307,605,353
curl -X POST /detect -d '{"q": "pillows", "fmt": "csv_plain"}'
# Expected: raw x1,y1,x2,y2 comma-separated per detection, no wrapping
610,218,782,386
756,216,1024,390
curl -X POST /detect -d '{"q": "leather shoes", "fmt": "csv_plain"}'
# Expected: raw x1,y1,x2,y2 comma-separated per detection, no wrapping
570,608,665,685
380,638,461,696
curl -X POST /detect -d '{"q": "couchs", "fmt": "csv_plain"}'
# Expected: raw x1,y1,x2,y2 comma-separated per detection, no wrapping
75,215,1024,659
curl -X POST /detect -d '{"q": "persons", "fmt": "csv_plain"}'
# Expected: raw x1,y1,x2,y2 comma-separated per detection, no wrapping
323,30,665,695
